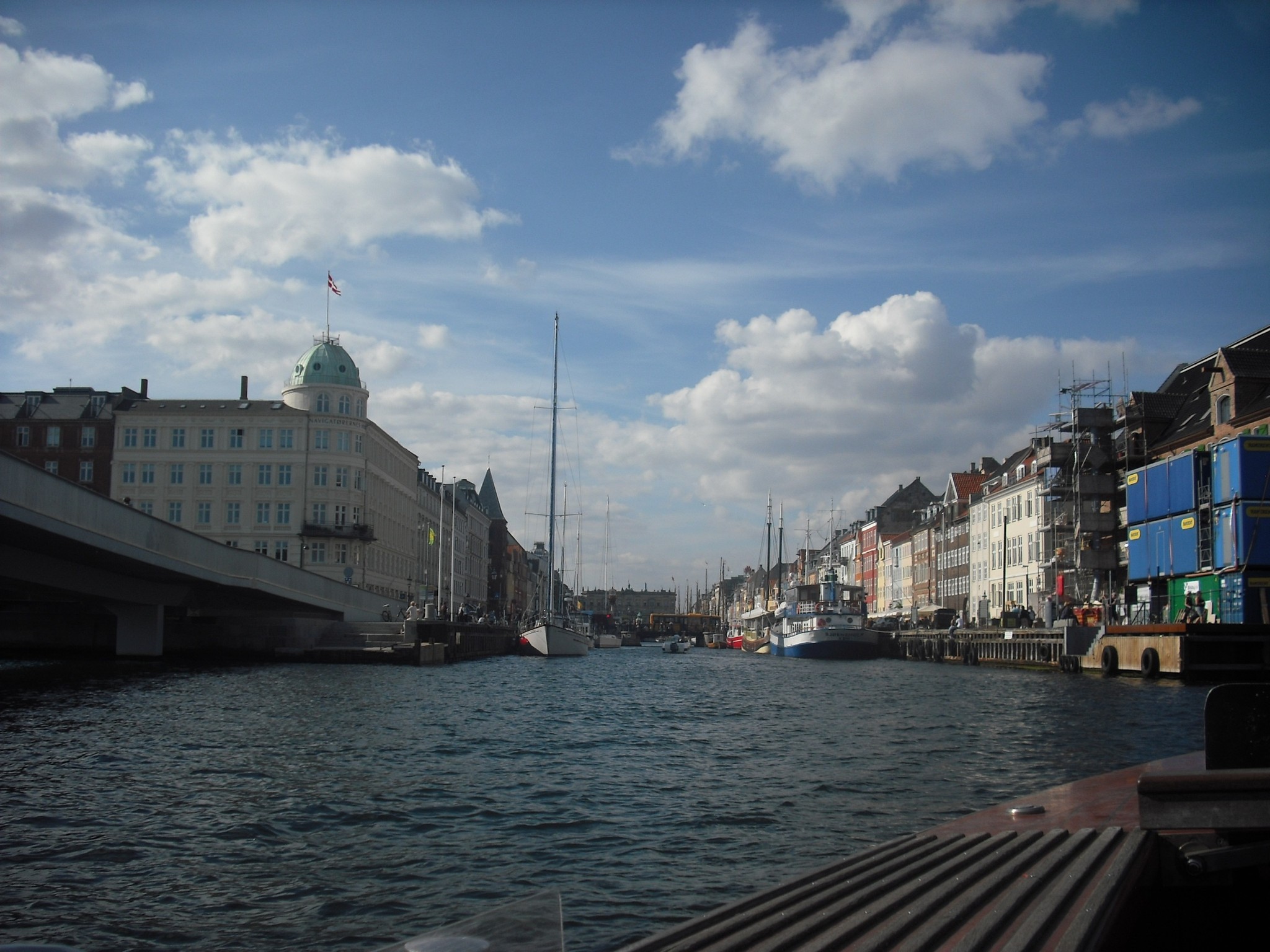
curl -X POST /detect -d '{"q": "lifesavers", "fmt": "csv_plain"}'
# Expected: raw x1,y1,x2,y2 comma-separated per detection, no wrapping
1058,654,1079,673
1140,647,1160,679
1099,645,1118,675
815,603,826,613
851,603,860,614
906,639,977,665
1038,644,1050,660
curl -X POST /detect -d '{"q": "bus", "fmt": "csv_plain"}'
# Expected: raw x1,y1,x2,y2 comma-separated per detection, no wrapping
649,612,723,633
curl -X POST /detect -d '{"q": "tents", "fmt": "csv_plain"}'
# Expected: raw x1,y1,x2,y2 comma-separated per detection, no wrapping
919,605,956,629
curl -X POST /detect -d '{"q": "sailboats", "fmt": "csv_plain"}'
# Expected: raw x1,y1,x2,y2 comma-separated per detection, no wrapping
570,492,642,649
515,310,590,656
736,488,791,654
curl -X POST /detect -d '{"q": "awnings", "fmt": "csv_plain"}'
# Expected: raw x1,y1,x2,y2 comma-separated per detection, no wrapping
867,606,928,618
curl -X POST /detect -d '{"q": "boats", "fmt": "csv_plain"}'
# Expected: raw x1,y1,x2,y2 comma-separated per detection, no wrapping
768,497,881,659
707,633,727,649
662,631,691,653
727,620,744,649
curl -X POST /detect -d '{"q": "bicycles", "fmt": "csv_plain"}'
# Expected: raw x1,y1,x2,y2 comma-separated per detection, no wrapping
381,603,392,623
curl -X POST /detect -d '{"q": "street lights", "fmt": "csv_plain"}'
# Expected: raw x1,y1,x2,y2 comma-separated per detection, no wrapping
299,542,310,569
492,569,497,613
405,573,413,608
1021,565,1030,610
465,593,471,622
445,586,450,608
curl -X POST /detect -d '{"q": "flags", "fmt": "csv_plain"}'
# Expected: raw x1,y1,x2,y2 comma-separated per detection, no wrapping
428,528,436,546
328,273,341,295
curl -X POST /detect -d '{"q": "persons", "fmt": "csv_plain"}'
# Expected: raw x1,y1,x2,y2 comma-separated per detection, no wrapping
965,618,976,629
458,603,466,615
1184,590,1193,624
1058,601,1080,626
402,601,420,634
437,603,447,615
1100,594,1119,626
948,615,962,639
1012,604,1036,629
123,497,132,507
900,613,933,631
1193,591,1205,624
476,603,495,624
